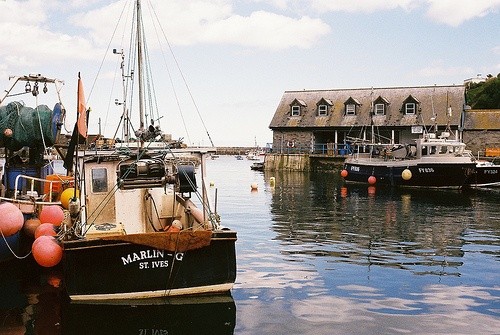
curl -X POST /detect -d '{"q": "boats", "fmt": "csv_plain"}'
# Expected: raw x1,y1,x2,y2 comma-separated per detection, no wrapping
0,0,243,304
250,161,264,172
340,83,500,190
245,144,266,160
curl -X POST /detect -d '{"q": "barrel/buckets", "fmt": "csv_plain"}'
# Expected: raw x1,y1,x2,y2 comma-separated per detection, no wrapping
8,168,36,189
